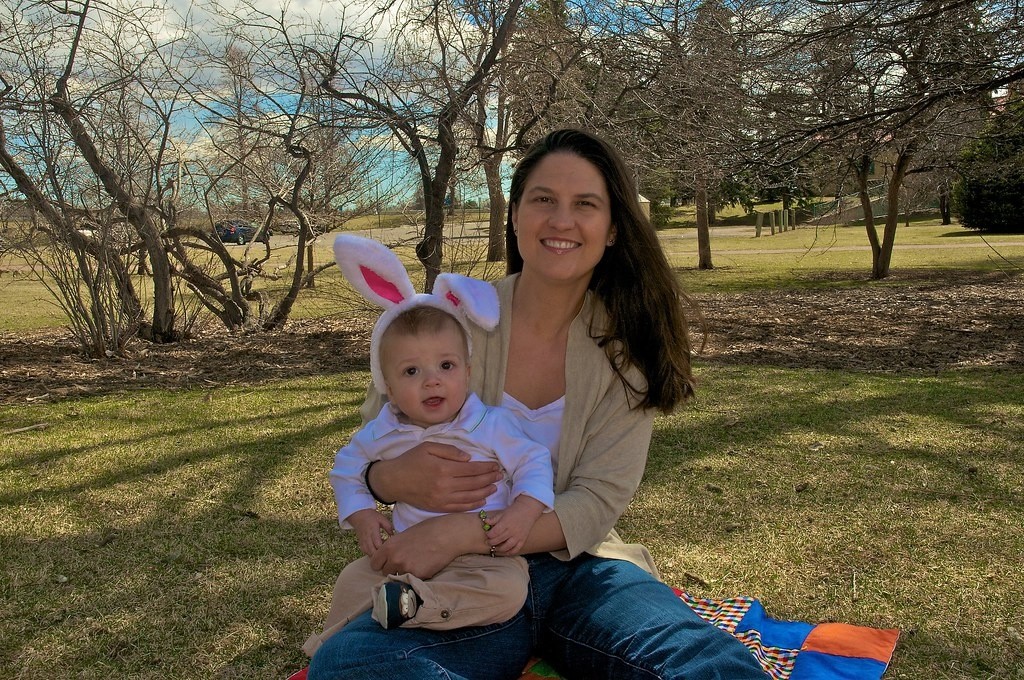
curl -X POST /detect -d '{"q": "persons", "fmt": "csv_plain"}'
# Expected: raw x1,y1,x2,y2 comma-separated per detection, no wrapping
300,232,557,660
306,129,777,680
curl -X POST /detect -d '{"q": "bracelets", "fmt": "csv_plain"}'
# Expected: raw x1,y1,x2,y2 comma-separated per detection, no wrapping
479,509,498,558
364,459,398,505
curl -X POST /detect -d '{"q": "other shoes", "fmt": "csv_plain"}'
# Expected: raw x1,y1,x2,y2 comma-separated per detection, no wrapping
376,582,418,629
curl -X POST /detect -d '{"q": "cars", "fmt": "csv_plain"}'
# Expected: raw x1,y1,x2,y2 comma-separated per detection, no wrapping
280,219,302,235
213,219,270,246
